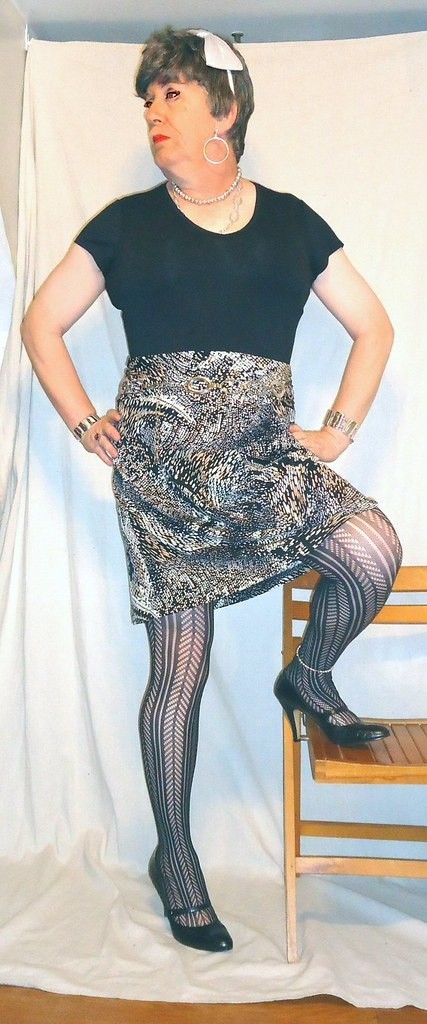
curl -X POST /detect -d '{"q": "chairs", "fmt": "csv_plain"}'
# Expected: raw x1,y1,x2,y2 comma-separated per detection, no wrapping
284,566,427,965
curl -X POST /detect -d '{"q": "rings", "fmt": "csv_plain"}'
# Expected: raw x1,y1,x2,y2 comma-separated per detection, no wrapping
94,433,101,441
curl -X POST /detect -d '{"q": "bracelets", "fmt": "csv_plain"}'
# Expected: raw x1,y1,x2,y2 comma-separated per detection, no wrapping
72,413,102,442
321,409,358,444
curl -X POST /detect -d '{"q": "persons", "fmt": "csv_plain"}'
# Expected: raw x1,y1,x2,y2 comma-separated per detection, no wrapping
20,27,402,952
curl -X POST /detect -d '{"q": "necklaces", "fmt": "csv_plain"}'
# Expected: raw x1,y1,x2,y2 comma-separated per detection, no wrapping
171,164,243,234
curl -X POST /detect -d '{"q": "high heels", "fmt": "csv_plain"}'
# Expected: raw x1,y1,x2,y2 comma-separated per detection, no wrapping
273,668,391,746
147,845,233,952
